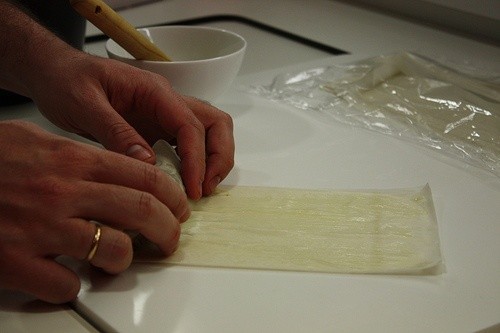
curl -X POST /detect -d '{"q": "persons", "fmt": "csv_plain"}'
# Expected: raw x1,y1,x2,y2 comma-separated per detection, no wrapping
0,0,235,303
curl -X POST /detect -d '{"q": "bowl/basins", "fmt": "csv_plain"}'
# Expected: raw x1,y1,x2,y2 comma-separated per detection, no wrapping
105,25,247,106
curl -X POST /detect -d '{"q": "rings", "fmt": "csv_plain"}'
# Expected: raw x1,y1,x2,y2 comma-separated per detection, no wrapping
85,220,101,261
